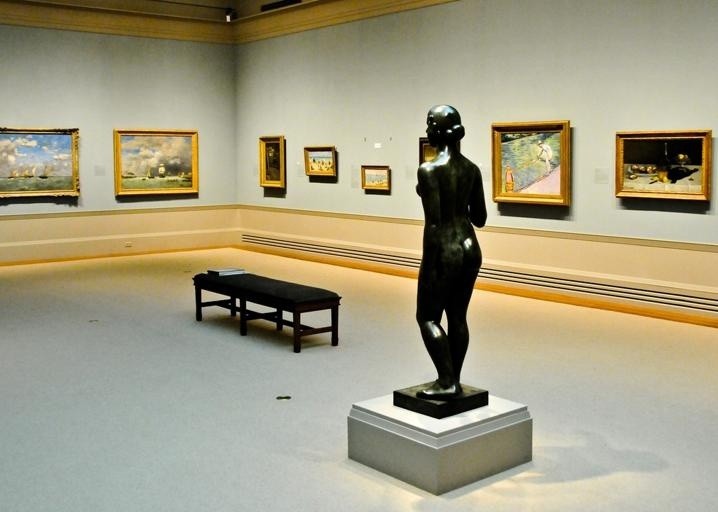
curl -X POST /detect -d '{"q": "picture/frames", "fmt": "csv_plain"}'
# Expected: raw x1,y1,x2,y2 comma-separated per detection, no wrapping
1,127,81,199
113,129,199,197
259,136,286,189
419,136,460,167
615,129,713,202
304,146,336,177
361,165,390,191
491,120,570,206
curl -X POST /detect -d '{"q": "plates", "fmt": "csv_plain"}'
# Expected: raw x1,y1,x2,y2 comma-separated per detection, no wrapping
627,168,658,177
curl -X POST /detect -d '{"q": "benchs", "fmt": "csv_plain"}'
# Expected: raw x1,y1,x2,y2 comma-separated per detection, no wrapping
193,272,341,353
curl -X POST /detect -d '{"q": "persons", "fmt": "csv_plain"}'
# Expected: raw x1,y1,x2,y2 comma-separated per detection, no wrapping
536,140,553,177
414,104,488,398
159,163,166,177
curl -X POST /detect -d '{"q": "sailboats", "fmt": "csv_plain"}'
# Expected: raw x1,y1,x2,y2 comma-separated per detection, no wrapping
122,163,193,183
7,165,53,179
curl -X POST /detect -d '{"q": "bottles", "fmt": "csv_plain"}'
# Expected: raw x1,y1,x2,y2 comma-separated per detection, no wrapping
657,142,671,183
504,162,514,192
